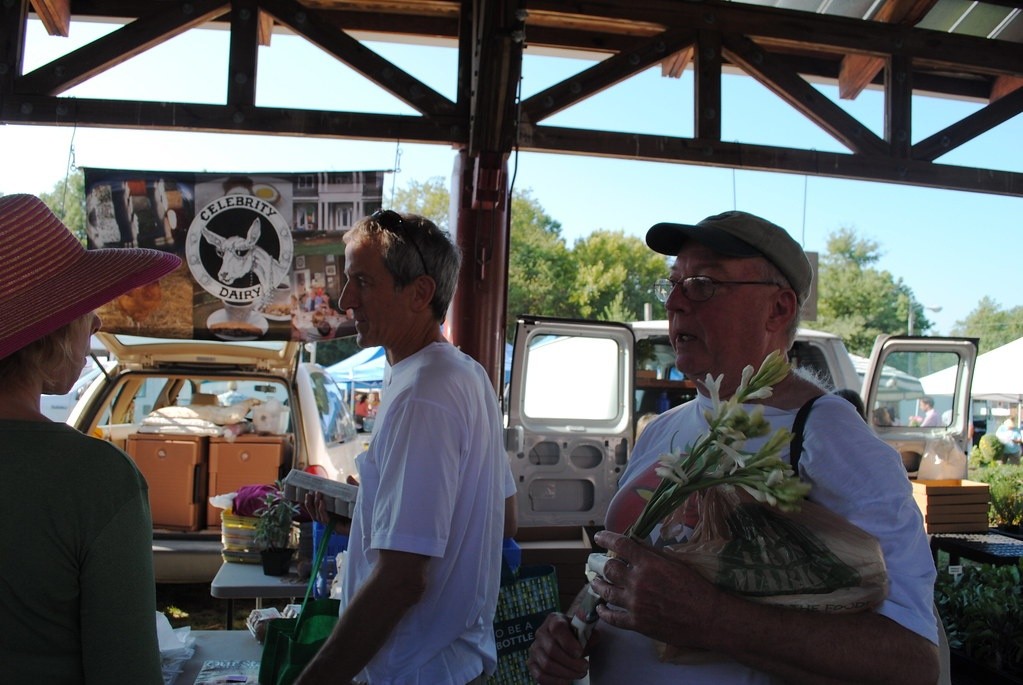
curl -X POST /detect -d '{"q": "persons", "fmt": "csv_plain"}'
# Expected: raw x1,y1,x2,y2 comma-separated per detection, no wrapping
871,395,1023,471
1,192,187,685
525,209,952,685
289,287,356,340
288,205,520,680
353,393,369,432
369,391,382,417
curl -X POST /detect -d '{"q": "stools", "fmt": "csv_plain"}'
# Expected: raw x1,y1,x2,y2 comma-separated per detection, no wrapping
931,527,1022,587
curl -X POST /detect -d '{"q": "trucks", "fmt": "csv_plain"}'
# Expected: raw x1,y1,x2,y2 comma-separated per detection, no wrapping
507,309,986,549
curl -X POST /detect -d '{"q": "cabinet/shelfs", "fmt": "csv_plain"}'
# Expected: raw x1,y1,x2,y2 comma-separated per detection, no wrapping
126,431,209,532
204,433,284,532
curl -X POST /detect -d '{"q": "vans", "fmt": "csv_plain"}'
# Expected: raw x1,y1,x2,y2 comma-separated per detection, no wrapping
56,319,368,605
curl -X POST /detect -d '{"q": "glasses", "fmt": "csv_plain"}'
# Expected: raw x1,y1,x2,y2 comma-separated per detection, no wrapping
367,208,441,299
651,272,787,304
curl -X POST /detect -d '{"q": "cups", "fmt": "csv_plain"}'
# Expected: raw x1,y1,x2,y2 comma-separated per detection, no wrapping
223,300,253,325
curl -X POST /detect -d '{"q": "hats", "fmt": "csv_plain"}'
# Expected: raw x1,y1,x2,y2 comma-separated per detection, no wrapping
645,209,811,306
0,193,185,367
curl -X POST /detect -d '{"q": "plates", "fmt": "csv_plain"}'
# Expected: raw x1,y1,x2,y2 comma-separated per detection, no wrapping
255,309,293,322
206,307,270,342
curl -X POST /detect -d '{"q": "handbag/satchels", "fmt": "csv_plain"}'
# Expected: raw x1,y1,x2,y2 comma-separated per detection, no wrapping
917,434,968,484
257,513,352,684
650,478,893,662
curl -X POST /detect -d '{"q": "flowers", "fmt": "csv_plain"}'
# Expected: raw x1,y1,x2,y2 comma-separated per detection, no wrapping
570,347,815,652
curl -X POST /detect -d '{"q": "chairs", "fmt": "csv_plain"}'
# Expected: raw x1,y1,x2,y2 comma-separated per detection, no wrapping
187,392,220,407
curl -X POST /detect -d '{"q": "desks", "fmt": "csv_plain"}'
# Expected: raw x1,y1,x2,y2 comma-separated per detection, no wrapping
207,560,319,632
